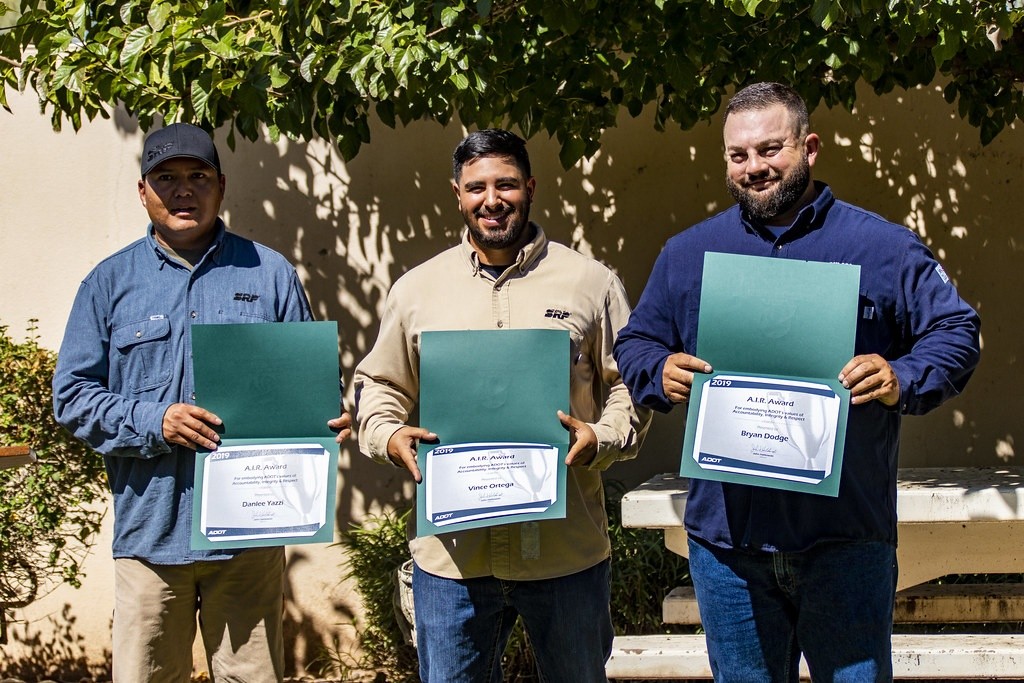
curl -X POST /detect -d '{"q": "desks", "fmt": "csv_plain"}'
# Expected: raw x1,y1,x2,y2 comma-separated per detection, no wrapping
619,464,1024,595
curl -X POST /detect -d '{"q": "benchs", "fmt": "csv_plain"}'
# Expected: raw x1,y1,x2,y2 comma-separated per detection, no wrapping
601,635,1024,683
662,582,1024,624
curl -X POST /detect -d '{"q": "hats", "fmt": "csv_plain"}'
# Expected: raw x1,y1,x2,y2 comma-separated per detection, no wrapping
141,122,223,183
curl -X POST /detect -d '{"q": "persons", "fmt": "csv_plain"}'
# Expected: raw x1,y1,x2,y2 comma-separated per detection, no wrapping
52,119,350,683
610,81,984,683
351,129,653,683
